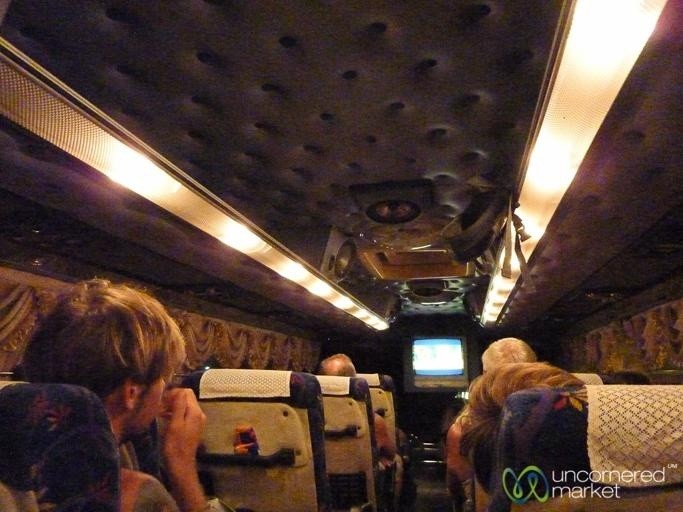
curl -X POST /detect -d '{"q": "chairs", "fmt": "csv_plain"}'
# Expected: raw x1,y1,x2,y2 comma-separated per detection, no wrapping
489,383,682,512
172,368,331,512
615,369,683,386
0,380,120,511
356,372,398,512
569,371,617,386
313,375,379,512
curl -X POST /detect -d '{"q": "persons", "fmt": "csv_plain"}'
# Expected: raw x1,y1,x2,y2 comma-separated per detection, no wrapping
445,337,537,499
20,280,234,512
460,361,585,492
316,353,397,468
610,373,653,384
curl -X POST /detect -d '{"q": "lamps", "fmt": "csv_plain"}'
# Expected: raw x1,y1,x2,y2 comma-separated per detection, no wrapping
477,0,667,334
0,36,391,333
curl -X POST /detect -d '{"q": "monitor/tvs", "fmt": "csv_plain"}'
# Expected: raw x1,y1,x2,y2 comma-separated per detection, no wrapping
411,336,469,389
